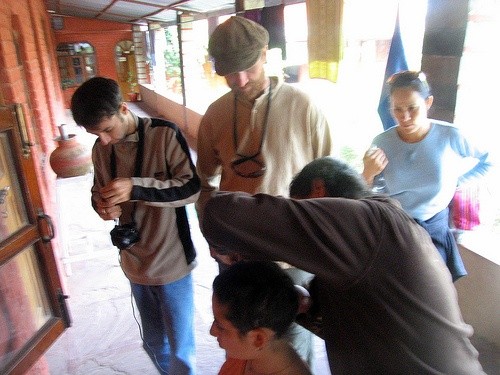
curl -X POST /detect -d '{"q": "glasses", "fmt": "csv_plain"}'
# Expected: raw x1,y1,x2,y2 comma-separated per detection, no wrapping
230,151,266,179
386,70,430,90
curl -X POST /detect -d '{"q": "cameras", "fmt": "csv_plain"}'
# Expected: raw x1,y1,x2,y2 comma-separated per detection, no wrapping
110,225,141,250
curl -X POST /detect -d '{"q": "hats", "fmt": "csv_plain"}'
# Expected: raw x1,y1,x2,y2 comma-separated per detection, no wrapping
207,15,269,75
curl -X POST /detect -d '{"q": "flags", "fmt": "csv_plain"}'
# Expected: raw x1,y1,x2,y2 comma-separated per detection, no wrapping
378,17,410,131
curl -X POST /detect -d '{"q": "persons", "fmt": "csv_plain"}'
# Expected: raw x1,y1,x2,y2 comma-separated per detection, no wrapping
209,258,316,375
361,70,495,283
69,76,202,375
199,157,488,375
77,46,90,64
195,15,331,375
